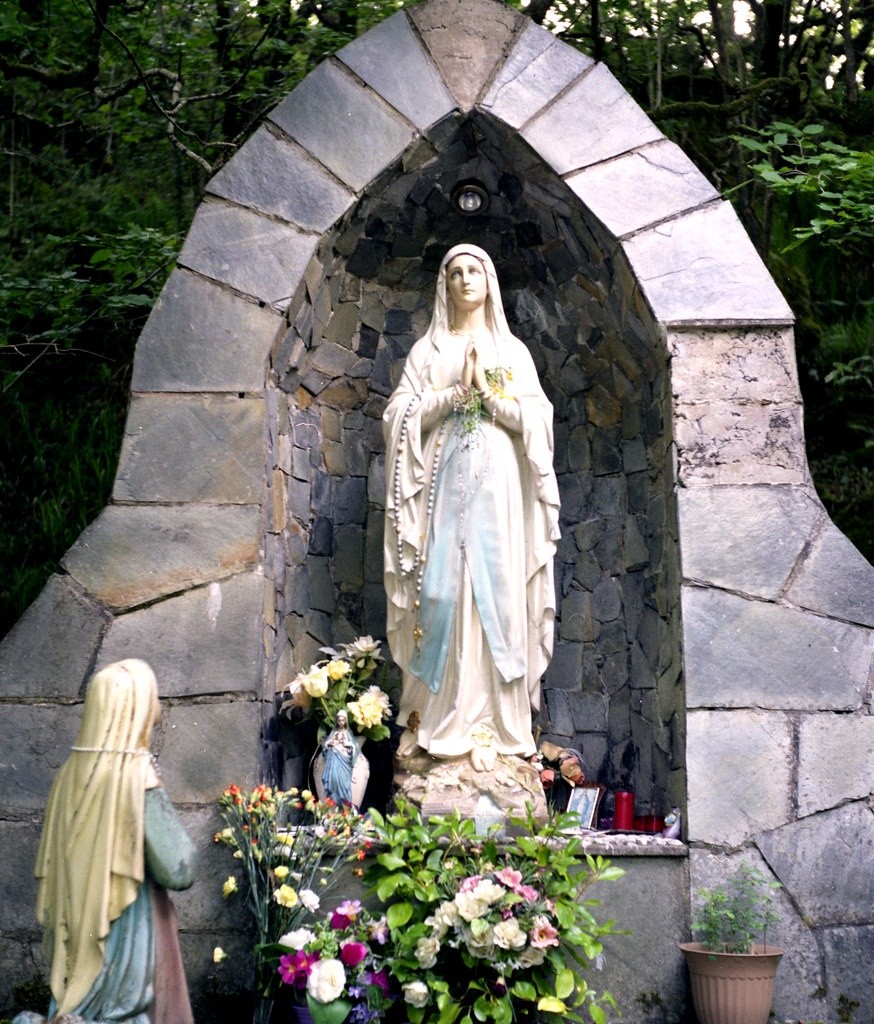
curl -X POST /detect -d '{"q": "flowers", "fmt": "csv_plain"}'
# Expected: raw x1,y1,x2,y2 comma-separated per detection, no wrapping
214,740,626,1024
459,365,514,434
275,634,395,745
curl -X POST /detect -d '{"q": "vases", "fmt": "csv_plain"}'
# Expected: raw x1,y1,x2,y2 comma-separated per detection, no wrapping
311,735,371,817
293,1004,314,1024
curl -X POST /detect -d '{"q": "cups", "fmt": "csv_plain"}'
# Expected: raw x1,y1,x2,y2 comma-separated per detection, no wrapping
635,814,663,833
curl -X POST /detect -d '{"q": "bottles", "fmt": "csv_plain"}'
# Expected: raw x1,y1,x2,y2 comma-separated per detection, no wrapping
614,784,634,830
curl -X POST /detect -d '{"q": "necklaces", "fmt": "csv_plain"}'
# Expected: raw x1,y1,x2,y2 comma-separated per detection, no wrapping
394,391,448,659
70,744,163,779
451,374,499,559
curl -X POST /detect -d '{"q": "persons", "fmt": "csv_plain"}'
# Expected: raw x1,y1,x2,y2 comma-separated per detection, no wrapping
33,658,199,1024
382,241,559,773
322,709,361,812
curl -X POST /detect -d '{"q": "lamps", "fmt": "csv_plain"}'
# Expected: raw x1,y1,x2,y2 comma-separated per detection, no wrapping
451,184,491,218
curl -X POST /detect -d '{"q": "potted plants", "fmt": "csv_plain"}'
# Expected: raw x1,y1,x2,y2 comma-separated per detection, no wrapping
678,869,784,1024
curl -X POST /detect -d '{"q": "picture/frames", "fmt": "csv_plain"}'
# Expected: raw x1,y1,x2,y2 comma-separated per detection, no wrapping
559,779,606,830
